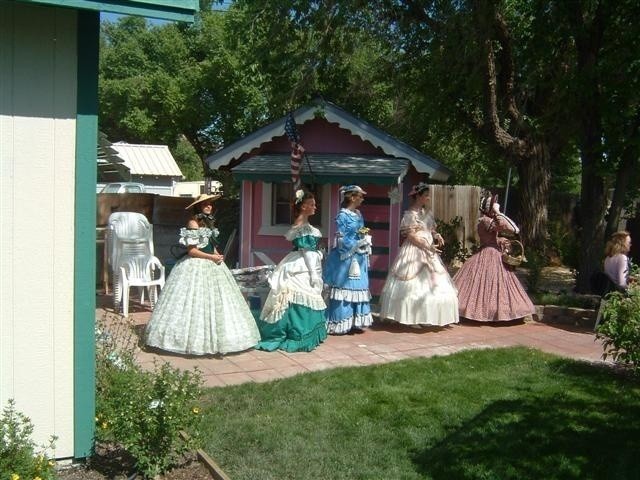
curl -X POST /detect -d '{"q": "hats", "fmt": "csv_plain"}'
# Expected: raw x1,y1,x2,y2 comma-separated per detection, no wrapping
407,185,429,196
341,185,368,194
479,194,498,216
185,194,221,212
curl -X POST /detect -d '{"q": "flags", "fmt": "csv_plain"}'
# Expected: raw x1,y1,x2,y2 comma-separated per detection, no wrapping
284,112,306,190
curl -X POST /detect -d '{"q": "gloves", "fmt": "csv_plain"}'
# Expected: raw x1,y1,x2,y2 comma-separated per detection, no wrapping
302,250,323,287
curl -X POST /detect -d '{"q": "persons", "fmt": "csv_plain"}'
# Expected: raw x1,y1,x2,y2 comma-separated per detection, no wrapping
143,192,261,357
380,182,462,326
452,194,536,322
603,228,637,290
254,188,328,353
320,185,375,335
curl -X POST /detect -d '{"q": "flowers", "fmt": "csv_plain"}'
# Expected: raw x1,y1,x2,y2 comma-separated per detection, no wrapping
359,227,372,269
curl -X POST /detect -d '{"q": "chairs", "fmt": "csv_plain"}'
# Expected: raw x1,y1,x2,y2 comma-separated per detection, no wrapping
106,211,166,319
